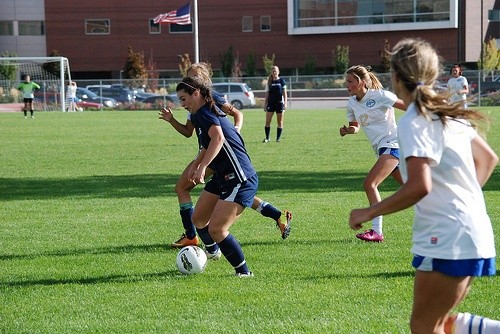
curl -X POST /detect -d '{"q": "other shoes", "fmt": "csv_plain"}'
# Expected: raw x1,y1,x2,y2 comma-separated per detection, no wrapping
276,138,280,142
31,116,35,118
262,139,270,143
24,116,27,118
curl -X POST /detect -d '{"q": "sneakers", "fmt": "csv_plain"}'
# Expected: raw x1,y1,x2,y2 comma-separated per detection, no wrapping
205,250,222,260
274,210,292,240
355,229,384,243
171,235,199,248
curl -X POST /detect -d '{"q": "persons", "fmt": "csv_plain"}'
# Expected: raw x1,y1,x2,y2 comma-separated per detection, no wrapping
340,66,408,243
447,64,469,108
349,39,500,334
159,61,292,248
176,76,258,279
68,81,78,111
17,75,41,118
262,66,287,142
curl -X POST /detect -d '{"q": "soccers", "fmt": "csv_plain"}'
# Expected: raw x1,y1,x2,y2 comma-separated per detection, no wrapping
176,246,207,275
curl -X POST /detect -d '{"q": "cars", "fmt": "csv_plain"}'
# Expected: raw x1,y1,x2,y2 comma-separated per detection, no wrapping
212,83,256,110
86,85,120,97
35,92,100,111
56,86,117,109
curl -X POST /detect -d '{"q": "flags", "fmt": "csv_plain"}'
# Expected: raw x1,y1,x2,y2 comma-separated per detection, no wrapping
153,2,191,25
